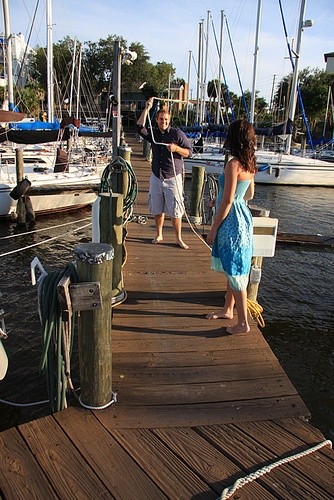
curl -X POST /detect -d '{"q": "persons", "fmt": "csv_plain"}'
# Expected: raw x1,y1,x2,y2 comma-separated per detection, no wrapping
134,99,192,250
206,119,257,335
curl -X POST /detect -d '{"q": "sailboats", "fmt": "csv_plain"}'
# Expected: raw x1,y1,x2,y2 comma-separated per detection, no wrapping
0,0,113,218
167,0,334,186
